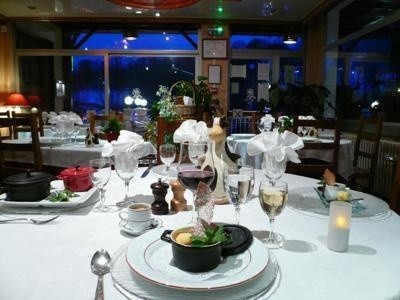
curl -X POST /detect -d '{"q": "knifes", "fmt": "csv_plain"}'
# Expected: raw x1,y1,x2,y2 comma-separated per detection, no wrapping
141,164,153,178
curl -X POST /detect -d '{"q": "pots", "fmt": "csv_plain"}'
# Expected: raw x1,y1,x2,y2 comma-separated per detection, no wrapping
57,164,95,191
5,169,54,201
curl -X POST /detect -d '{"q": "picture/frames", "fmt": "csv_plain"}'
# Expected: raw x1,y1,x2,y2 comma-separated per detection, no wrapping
202,37,228,61
207,64,222,85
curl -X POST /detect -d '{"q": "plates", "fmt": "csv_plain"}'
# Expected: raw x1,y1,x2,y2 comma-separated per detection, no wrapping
117,216,165,235
284,188,390,218
0,188,98,207
299,134,336,142
153,163,196,177
108,222,279,300
231,133,255,139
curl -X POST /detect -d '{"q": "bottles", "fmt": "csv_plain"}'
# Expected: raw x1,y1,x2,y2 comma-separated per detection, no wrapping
85,129,93,146
151,177,187,214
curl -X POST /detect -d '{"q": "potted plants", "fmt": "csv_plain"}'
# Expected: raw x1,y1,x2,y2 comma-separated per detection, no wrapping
101,117,123,141
175,79,213,114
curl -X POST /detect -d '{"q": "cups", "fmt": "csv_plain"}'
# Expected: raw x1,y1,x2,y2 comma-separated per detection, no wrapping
119,203,153,229
326,199,353,252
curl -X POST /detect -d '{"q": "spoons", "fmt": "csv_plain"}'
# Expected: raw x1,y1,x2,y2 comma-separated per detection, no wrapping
90,249,111,300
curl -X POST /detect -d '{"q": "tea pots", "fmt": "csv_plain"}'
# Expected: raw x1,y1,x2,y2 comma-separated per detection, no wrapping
202,116,240,203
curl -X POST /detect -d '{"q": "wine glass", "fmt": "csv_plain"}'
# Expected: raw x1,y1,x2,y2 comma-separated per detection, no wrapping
178,143,215,225
40,110,82,147
160,144,177,174
258,118,310,140
258,149,290,249
224,164,254,224
88,150,138,210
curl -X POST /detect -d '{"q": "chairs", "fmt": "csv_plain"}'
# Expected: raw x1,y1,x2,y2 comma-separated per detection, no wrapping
0,109,53,173
285,113,386,192
155,112,211,164
89,112,125,139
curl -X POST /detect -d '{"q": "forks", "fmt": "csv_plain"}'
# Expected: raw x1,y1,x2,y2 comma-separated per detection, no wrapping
0,215,60,225
